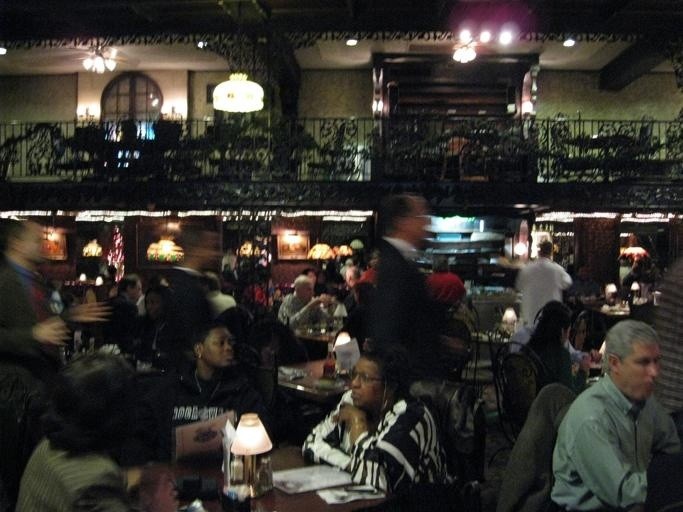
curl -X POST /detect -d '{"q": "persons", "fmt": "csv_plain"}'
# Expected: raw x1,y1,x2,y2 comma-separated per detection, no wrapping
1,193,682,511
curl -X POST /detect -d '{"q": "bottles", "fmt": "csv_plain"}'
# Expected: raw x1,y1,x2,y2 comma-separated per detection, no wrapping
322,341,336,377
230,453,273,492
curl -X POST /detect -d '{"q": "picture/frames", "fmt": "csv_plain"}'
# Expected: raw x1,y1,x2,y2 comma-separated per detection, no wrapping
277,234,311,261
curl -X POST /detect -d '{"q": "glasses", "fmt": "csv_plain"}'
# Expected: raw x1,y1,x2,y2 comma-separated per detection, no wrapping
348,368,382,384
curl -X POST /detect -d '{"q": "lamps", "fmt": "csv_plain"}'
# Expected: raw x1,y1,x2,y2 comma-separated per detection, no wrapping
212,0,264,113
82,37,119,75
146,216,185,262
